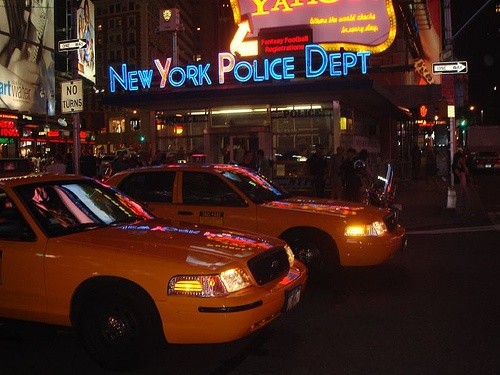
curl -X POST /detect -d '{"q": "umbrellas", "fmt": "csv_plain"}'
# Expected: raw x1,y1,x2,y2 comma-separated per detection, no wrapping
378,163,394,193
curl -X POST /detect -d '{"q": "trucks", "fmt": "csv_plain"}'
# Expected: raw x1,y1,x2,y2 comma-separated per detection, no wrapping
467,126,500,155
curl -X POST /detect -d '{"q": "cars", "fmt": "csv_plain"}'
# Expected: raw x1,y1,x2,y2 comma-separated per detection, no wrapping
0,157,308,367
88,153,408,285
470,150,500,173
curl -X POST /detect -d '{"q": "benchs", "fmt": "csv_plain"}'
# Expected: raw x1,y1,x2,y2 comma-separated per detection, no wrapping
285,175,318,196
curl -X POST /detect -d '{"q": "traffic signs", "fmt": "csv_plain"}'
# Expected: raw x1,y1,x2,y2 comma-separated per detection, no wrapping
57,39,86,53
431,60,470,75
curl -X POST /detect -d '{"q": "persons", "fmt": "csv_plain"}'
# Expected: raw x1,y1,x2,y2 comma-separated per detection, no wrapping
161,141,198,163
453,152,469,214
80,146,147,179
242,149,271,180
78,0,94,67
0,0,53,113
286,143,375,204
28,152,72,174
